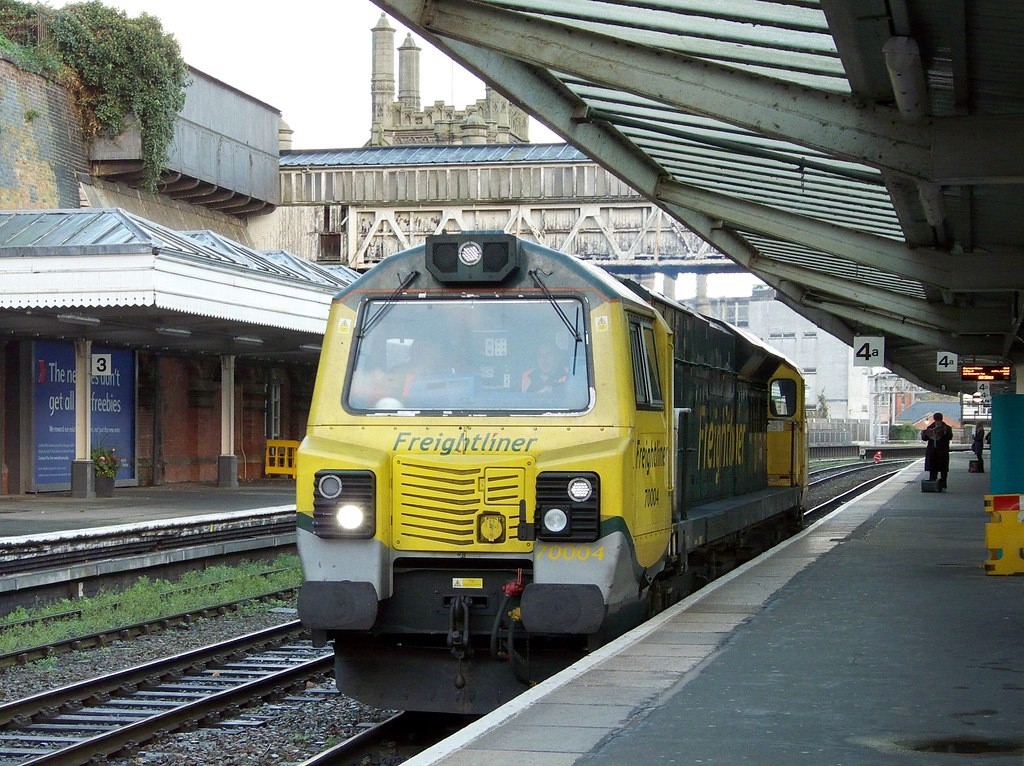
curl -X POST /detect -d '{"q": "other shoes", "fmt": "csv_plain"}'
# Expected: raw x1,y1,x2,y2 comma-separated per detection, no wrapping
939,486,947,492
977,468,984,473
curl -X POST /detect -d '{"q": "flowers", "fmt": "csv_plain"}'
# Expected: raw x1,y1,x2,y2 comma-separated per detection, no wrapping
92,431,122,478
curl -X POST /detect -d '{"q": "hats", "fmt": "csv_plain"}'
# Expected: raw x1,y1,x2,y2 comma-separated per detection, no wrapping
934,413,942,420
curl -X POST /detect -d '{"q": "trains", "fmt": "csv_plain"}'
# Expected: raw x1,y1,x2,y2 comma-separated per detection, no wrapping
295,231,810,714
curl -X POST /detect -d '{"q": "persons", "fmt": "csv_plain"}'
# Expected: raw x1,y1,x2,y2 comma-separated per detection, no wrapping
921,412,954,489
520,341,575,397
971,422,986,473
388,338,441,389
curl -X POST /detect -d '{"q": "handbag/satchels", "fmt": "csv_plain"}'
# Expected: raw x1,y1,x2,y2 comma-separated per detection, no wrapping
968,460,979,473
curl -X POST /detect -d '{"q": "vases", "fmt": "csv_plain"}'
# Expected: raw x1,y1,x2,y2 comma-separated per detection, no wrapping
95,475,115,497
